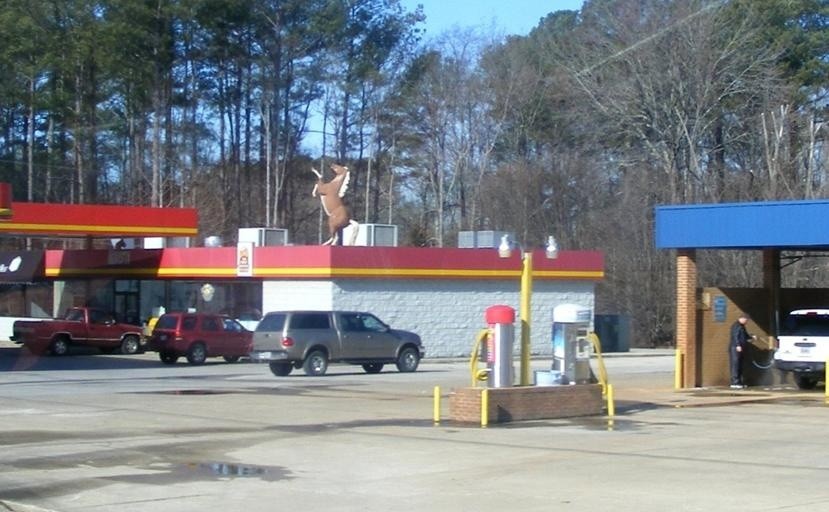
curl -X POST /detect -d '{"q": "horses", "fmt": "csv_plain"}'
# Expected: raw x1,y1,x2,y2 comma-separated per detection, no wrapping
307,163,360,247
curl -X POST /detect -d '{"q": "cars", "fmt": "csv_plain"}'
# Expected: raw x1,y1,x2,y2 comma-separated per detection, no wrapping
152,311,425,376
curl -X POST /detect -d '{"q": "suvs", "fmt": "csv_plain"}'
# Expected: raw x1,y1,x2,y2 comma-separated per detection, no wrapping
773,309,829,389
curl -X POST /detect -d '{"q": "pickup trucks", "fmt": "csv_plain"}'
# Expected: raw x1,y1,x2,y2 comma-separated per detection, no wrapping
10,307,148,356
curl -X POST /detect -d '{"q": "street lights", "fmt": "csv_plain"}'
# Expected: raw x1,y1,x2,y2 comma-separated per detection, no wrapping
498,234,559,386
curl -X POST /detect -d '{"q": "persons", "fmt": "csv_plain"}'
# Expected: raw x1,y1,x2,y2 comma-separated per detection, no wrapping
728,313,758,388
